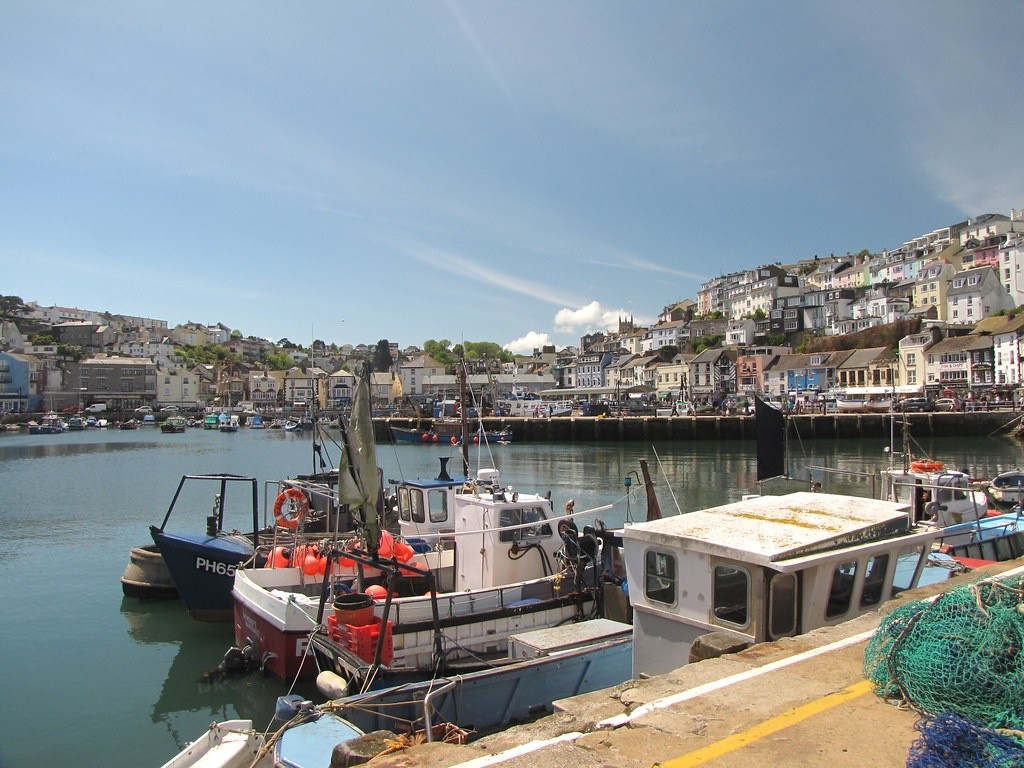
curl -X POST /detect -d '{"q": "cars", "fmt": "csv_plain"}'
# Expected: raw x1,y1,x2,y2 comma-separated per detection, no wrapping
134,405,153,413
160,405,179,412
934,398,959,411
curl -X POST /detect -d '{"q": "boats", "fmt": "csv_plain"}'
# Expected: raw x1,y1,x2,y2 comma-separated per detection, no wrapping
832,510,1024,595
991,466,1024,506
231,469,669,683
738,457,989,530
2,413,317,436
148,467,478,624
307,570,633,701
393,421,515,446
235,490,962,765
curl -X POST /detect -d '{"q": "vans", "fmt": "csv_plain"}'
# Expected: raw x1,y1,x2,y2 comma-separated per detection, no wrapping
85,403,107,413
893,396,934,412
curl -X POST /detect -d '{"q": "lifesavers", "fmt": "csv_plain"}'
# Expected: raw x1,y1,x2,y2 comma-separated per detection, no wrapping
274,490,309,530
912,460,944,474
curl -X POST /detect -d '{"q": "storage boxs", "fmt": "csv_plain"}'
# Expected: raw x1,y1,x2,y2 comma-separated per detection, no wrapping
326,614,394,667
